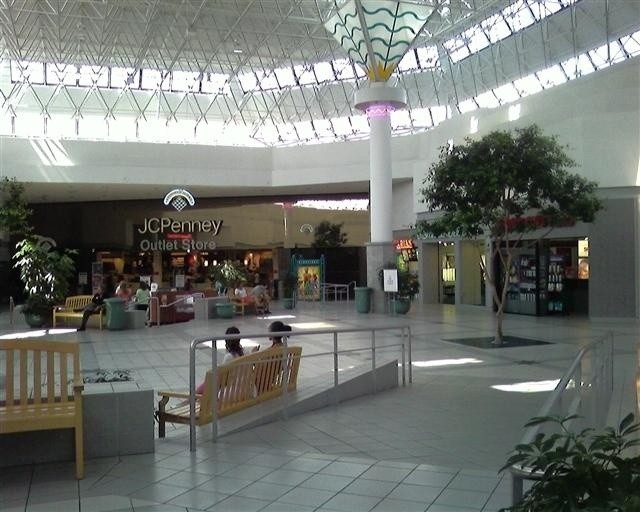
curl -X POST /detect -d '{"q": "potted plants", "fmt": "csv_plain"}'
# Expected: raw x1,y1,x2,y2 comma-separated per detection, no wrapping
12,239,79,328
279,272,297,309
376,263,418,314
207,263,244,318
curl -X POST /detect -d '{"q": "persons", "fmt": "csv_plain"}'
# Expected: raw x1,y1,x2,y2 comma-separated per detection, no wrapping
251,281,271,314
234,281,265,315
115,280,133,306
168,326,259,409
72,283,108,331
302,267,316,306
311,273,321,306
251,321,292,396
130,281,151,328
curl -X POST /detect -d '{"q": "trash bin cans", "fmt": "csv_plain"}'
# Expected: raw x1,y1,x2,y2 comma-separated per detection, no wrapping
354,287,371,313
104,298,126,330
278,281,285,299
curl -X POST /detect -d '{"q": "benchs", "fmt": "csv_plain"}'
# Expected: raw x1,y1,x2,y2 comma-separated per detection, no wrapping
53,295,107,332
0,339,85,481
229,287,269,316
155,347,302,438
